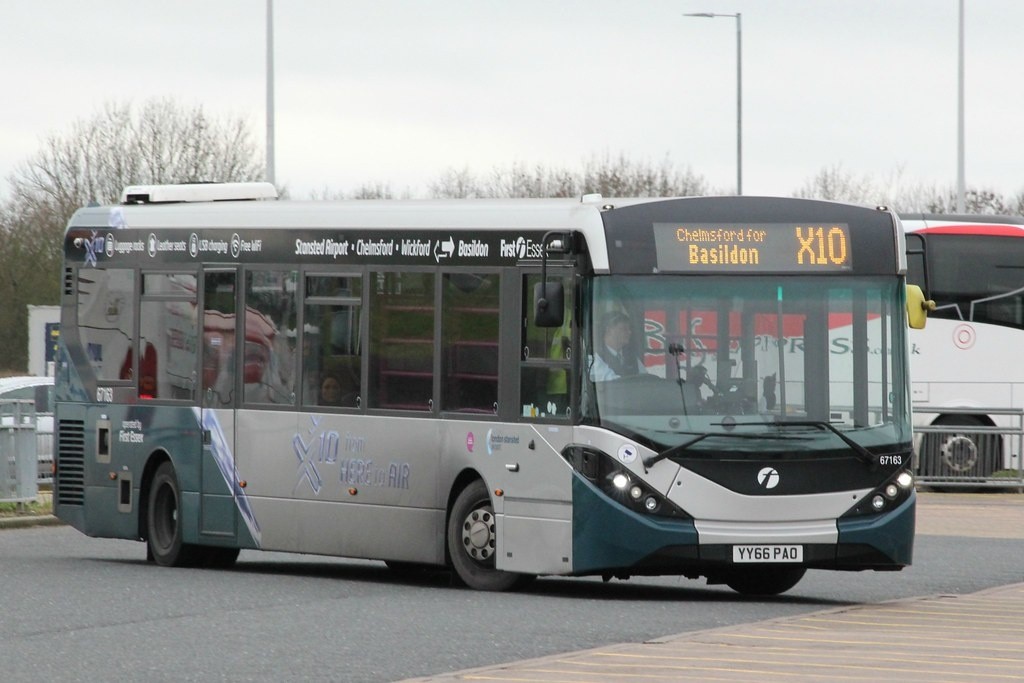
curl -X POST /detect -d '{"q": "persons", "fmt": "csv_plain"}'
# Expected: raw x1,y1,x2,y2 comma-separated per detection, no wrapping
310,374,344,406
586,309,647,382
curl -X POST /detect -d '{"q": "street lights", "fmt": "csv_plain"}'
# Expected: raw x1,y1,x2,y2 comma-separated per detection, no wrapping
681,10,743,196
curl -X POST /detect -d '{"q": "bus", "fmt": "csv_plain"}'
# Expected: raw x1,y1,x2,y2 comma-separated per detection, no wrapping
51,181,936,598
643,213,1024,491
